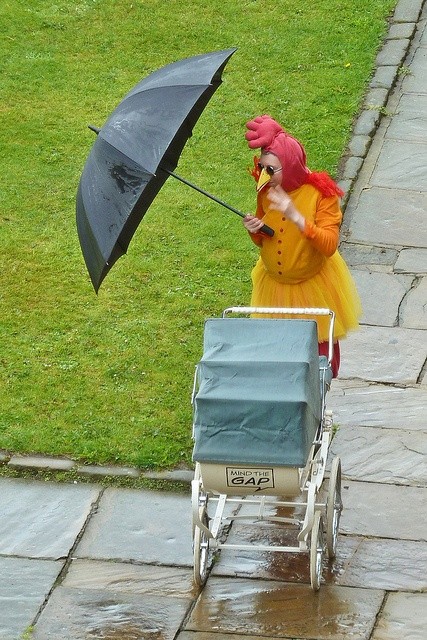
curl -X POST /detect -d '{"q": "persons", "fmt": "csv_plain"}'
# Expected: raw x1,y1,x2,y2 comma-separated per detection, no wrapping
246,115,363,378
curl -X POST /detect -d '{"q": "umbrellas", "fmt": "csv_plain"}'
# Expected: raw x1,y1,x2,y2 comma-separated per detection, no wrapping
75,48,275,295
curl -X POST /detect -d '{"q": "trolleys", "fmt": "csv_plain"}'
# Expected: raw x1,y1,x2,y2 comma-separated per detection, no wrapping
191,307,343,591
